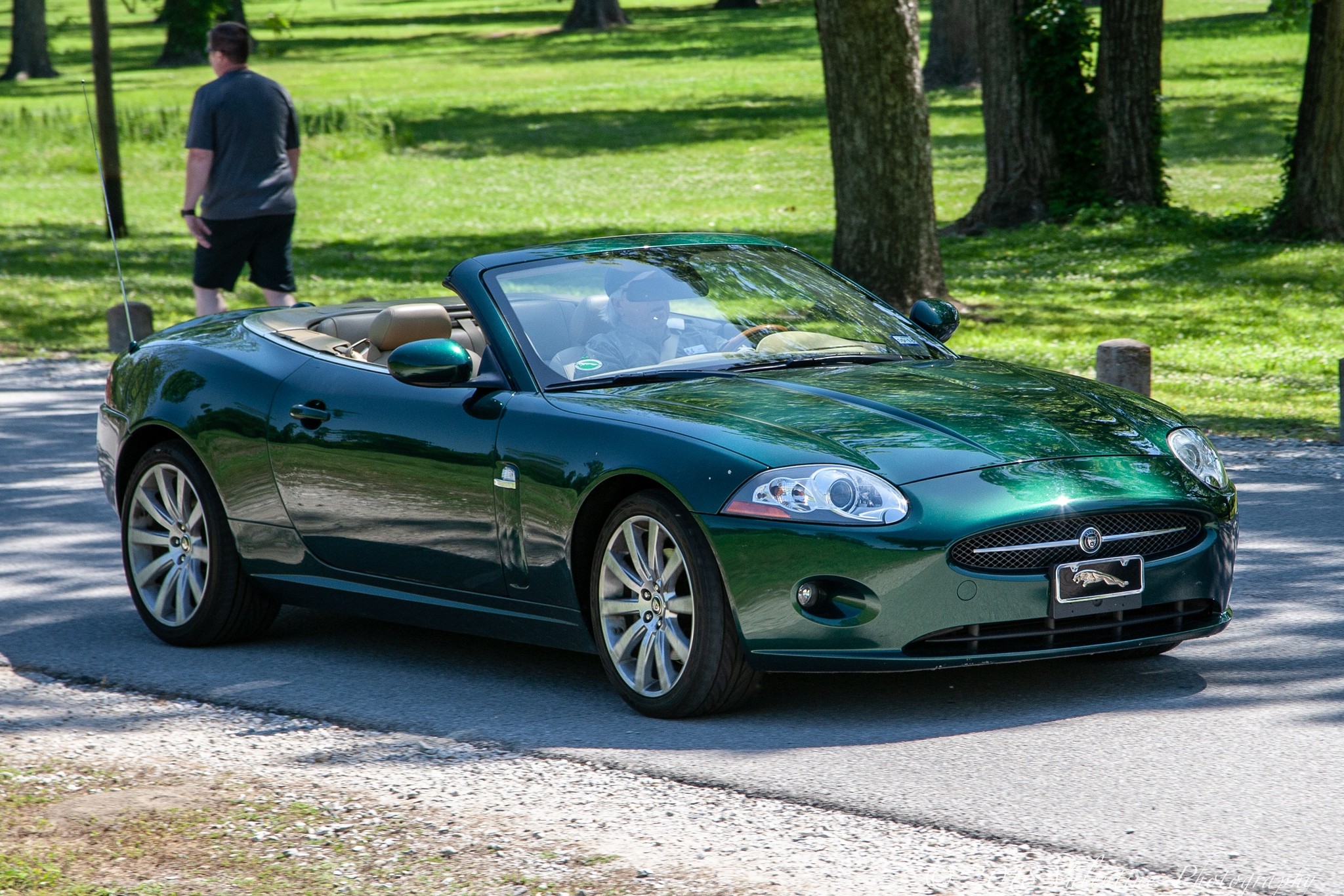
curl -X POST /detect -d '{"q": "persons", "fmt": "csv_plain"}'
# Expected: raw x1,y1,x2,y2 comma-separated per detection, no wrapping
575,266,729,379
184,22,301,317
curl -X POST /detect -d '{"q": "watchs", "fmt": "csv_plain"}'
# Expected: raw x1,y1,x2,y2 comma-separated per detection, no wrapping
180,209,196,218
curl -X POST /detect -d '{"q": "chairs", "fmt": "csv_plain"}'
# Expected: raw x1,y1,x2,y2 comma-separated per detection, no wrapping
358,303,483,378
548,292,615,381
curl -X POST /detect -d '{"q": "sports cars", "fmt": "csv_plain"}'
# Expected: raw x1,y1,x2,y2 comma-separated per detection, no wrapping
95,230,1238,722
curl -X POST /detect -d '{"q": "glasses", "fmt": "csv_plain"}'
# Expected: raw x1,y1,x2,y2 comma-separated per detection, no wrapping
202,44,225,58
